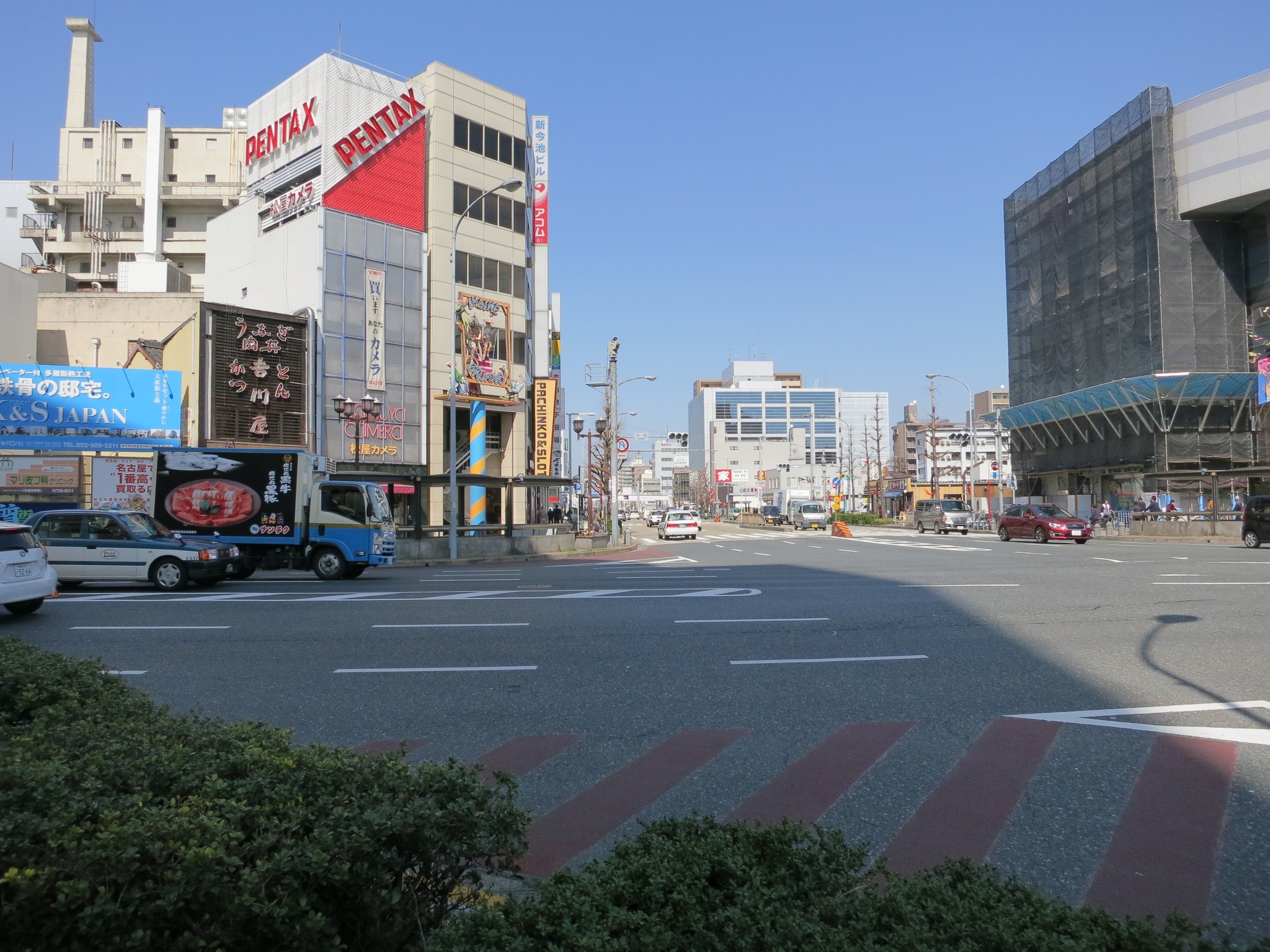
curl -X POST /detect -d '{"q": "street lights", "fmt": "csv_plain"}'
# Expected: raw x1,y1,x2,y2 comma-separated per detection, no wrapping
331,391,385,471
819,415,851,513
448,179,523,560
758,435,766,506
571,415,609,531
689,470,696,504
724,456,729,514
712,462,716,510
925,374,975,528
566,412,596,512
607,336,658,546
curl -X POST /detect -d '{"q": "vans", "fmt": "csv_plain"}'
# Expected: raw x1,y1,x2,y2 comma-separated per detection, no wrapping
760,489,826,531
914,499,971,535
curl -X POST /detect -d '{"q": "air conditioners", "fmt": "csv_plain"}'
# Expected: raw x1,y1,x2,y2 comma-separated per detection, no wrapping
941,455,945,459
976,440,980,444
946,470,952,474
981,440,986,444
946,440,951,444
940,440,945,444
981,455,986,459
977,455,980,459
946,455,951,459
941,470,946,474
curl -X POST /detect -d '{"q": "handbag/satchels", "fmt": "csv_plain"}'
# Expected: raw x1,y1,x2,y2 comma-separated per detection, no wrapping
1241,504,1244,511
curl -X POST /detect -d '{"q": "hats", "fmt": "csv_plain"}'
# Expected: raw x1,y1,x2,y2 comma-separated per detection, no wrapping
1235,496,1240,500
541,506,544,509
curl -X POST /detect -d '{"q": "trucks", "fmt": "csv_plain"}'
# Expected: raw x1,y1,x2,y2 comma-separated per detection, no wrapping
150,446,398,581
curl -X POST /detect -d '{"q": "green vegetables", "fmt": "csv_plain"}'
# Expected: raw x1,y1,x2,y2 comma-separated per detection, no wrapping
200,496,221,515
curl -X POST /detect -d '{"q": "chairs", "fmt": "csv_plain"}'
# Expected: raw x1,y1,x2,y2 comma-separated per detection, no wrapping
1026,508,1034,517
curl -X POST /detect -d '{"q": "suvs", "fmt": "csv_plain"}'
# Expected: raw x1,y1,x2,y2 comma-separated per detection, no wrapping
728,508,744,520
1241,494,1270,549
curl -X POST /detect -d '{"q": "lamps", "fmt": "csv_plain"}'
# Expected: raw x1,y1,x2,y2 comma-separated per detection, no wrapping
27,354,39,365
410,471,418,480
571,475,580,483
514,473,525,482
75,359,83,366
155,362,166,377
116,360,125,368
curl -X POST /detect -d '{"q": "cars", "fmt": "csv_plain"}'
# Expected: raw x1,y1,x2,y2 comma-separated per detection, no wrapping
847,503,872,514
997,503,1093,544
0,521,57,615
618,503,710,541
21,500,243,593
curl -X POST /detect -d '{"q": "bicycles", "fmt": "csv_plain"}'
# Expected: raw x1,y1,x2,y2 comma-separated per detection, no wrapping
1088,512,1121,531
1158,512,1186,522
972,509,1000,530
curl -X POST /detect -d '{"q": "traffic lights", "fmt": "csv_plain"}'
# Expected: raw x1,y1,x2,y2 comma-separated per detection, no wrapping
947,432,969,447
667,432,688,447
777,463,790,472
1011,475,1016,487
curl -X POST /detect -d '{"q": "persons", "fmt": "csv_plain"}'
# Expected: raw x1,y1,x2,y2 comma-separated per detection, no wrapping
327,488,356,518
1207,498,1214,511
592,508,602,533
1130,495,1162,521
1233,496,1243,521
1101,499,1115,522
537,504,573,525
100,520,129,541
703,509,709,519
1166,499,1178,521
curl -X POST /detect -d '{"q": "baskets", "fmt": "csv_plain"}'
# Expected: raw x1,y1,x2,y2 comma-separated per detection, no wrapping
1111,513,1118,517
